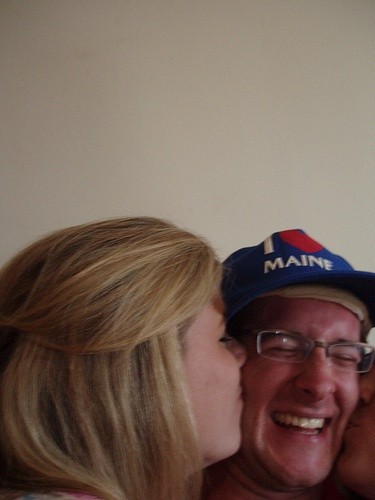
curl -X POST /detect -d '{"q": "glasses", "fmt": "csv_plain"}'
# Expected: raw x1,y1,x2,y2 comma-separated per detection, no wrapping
233,330,375,372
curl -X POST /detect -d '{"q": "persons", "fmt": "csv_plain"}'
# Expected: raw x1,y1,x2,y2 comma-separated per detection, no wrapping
201,229,375,500
0,216,249,500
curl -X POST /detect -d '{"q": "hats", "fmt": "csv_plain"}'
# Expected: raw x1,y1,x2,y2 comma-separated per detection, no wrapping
222,229,375,327
